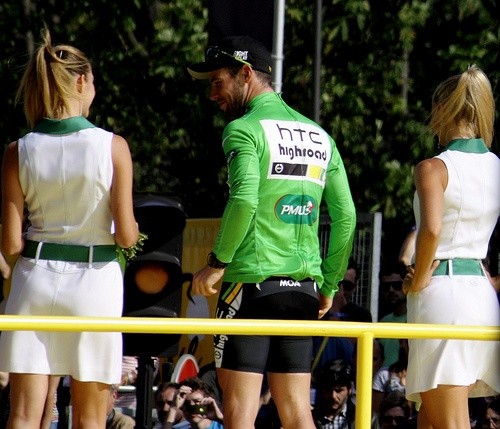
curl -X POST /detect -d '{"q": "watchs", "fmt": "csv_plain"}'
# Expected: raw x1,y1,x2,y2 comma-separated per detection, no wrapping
207,251,228,268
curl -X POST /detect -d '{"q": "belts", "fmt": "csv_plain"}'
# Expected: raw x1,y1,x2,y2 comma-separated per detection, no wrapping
22,240,117,262
432,259,485,275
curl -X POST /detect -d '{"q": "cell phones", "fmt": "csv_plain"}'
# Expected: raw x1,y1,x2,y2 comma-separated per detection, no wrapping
189,404,208,415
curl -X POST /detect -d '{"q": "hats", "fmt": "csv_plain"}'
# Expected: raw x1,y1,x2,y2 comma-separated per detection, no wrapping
188,34,274,79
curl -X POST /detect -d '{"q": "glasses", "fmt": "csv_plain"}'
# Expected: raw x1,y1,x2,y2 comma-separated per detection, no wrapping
337,280,355,291
381,280,402,291
204,45,254,71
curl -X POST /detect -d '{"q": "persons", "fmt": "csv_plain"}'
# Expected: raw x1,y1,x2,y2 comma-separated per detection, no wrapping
403,62,500,429
374,264,409,367
0,23,138,429
311,256,371,376
0,340,500,429
188,36,356,429
